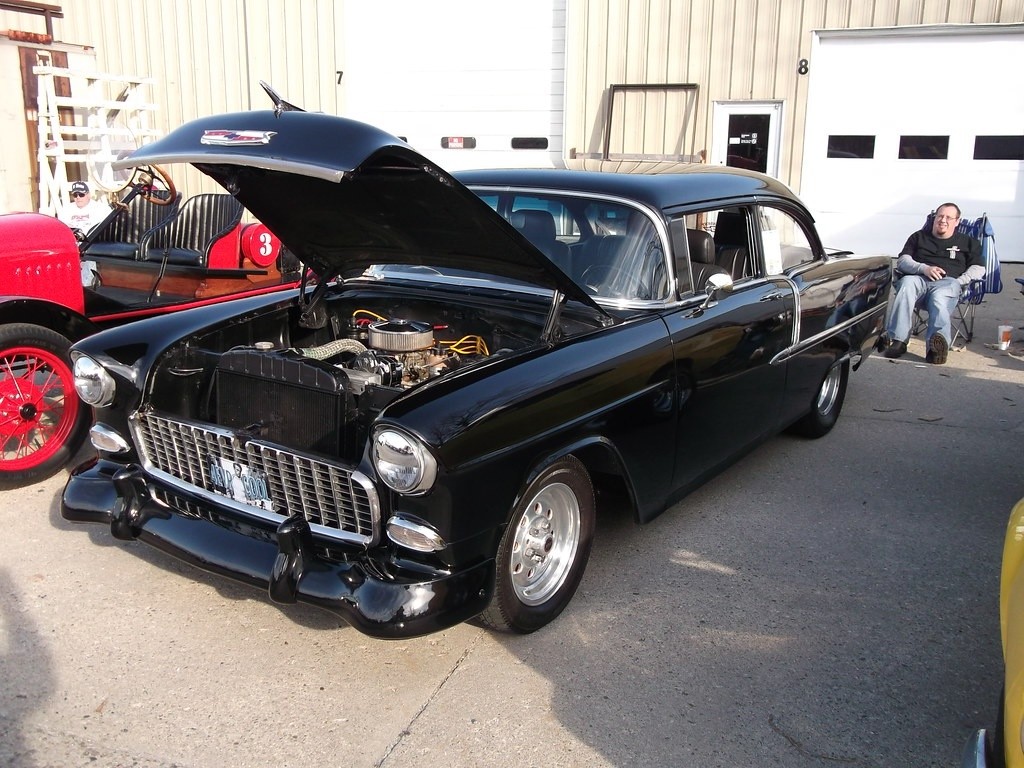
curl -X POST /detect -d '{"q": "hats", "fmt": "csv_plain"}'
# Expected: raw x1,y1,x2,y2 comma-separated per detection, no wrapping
72,181,90,194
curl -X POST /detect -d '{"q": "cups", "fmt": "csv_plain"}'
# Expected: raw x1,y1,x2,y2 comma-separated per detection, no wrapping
998,326,1013,351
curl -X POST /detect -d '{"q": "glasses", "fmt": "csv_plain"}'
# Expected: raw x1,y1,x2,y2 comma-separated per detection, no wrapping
72,192,86,198
933,215,957,221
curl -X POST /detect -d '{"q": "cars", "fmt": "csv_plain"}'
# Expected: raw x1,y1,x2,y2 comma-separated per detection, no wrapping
0,150,327,488
59,82,896,643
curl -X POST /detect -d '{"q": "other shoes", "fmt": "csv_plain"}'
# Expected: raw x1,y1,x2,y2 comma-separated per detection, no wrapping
929,333,948,364
885,340,907,358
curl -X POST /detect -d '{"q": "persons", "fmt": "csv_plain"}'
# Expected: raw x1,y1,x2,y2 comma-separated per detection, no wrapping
57,181,113,287
884,202,986,364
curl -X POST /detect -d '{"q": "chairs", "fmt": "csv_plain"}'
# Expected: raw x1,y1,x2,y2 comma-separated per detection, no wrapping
653,229,732,300
141,193,244,279
81,187,183,271
893,208,989,351
508,210,571,275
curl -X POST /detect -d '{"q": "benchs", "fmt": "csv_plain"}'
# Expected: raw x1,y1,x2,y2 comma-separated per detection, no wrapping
576,233,750,285
